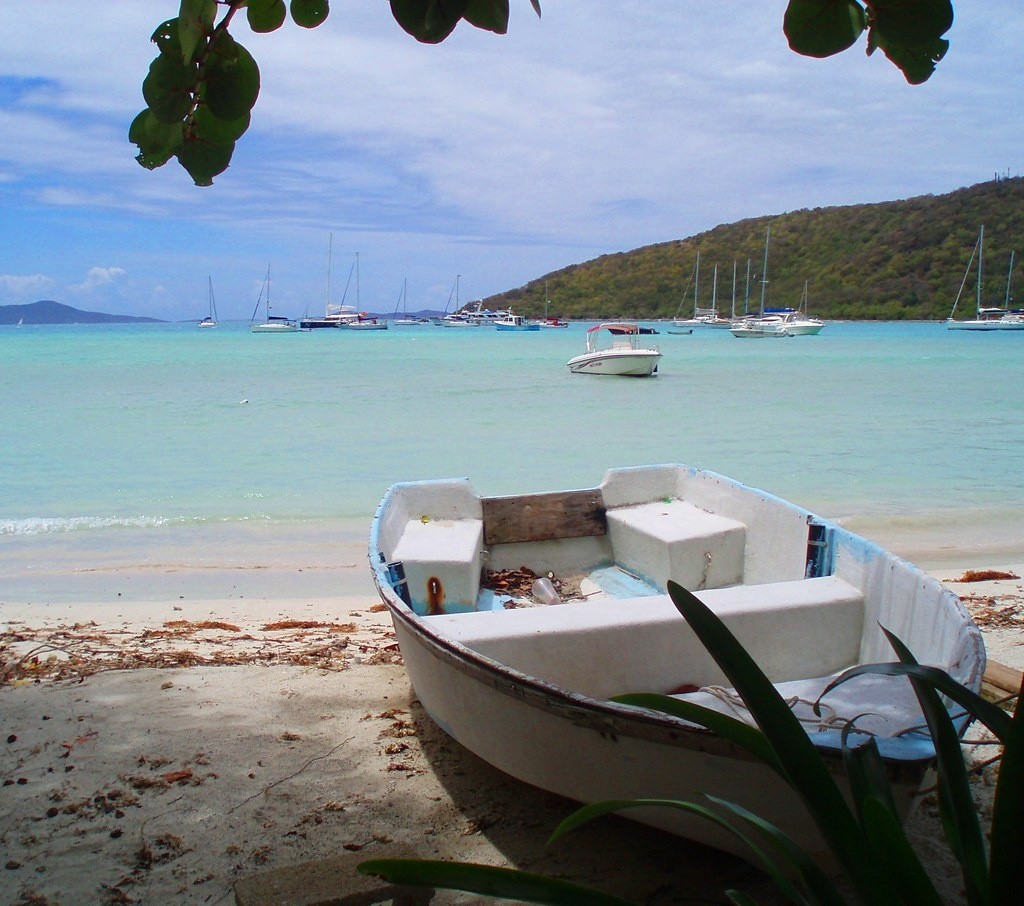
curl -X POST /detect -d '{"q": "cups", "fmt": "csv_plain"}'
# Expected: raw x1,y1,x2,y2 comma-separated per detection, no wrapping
532,579,561,605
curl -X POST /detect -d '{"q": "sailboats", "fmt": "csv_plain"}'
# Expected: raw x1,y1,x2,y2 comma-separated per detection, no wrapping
403,274,569,327
249,263,299,332
674,220,826,338
391,277,422,325
196,274,219,328
300,230,388,331
945,223,1024,330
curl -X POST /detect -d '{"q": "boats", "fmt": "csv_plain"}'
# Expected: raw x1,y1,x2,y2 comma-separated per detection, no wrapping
370,476,989,897
493,306,541,331
666,329,692,334
566,322,664,375
